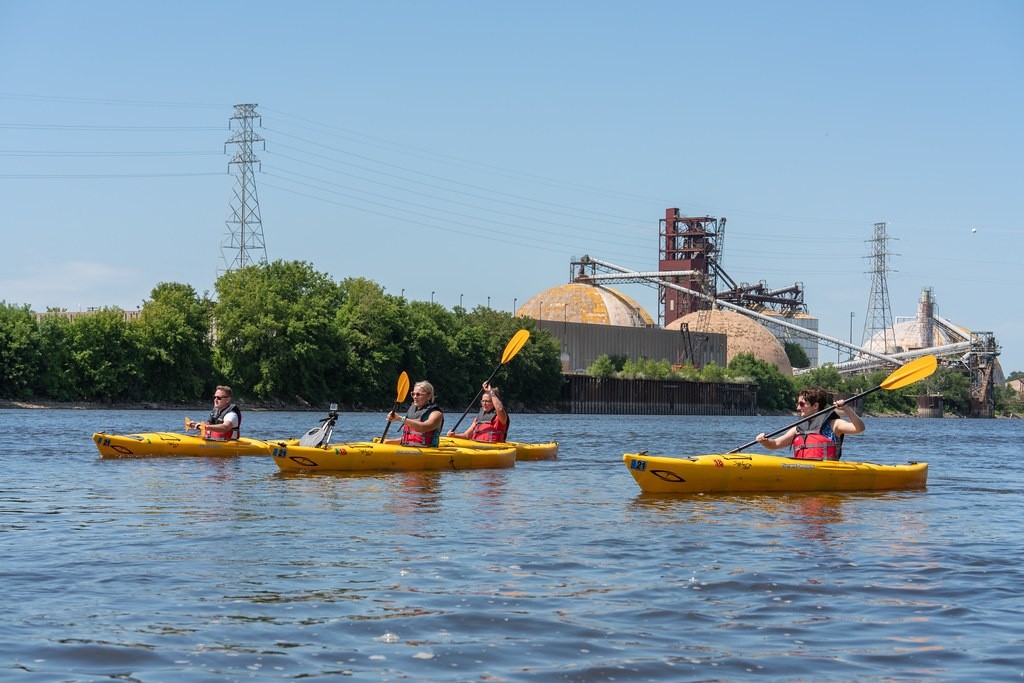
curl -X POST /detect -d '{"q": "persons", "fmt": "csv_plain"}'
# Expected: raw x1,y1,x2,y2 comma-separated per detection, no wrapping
383,380,444,451
187,385,242,442
759,375,866,464
445,381,511,447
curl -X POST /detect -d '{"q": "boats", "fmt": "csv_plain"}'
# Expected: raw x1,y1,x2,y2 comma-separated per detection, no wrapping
92,431,301,457
623,451,928,491
374,436,559,461
268,442,516,471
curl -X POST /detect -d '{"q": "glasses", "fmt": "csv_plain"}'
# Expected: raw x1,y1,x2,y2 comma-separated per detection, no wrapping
797,401,811,407
212,396,228,400
480,399,492,404
411,391,429,396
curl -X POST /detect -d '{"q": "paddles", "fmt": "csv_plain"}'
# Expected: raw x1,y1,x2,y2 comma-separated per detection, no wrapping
725,355,937,455
452,330,529,431
184,417,206,436
381,369,410,441
